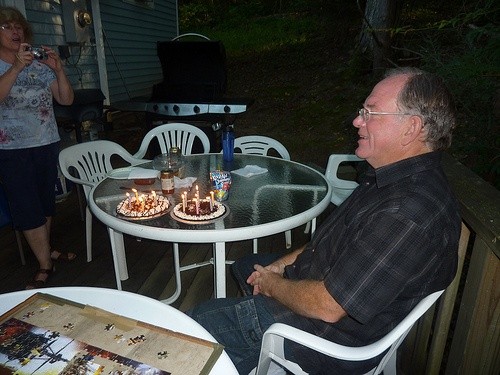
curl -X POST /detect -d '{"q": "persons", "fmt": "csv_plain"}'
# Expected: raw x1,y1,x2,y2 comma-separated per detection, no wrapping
185,62,461,375
0,6,79,289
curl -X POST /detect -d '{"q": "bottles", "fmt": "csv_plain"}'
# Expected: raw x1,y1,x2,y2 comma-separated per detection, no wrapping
170,147,180,164
161,170,174,194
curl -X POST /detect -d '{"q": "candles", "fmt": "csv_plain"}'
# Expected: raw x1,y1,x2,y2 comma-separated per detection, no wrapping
132,189,139,202
193,185,199,213
210,192,215,204
207,197,213,211
125,192,131,208
184,192,188,207
141,195,146,206
181,194,186,213
152,191,157,205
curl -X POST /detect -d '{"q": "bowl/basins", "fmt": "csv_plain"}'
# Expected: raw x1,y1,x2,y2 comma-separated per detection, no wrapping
152,153,192,180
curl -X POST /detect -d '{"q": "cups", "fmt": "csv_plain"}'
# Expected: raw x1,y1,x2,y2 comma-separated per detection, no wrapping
223,132,234,162
210,170,232,201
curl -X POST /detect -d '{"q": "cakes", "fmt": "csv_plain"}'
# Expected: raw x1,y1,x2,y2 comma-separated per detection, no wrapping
116,194,169,218
173,198,226,221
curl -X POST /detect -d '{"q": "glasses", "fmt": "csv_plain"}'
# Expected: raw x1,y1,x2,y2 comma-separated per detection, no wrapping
0,24,23,33
358,108,424,128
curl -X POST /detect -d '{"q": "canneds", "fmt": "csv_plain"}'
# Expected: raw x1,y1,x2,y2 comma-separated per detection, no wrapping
169,147,179,167
160,169,174,194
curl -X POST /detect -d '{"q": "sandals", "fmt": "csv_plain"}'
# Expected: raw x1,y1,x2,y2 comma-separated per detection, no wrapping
54,250,78,262
26,264,57,288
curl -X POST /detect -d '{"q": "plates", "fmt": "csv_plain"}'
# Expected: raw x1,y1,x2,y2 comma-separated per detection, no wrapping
170,199,230,224
117,196,175,220
106,167,144,179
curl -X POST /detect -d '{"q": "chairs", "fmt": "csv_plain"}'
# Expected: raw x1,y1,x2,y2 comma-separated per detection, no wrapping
58,138,155,280
304,153,366,235
132,123,210,163
251,287,446,375
219,135,291,255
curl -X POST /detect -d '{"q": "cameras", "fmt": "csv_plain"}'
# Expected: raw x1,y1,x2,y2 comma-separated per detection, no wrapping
26,46,44,60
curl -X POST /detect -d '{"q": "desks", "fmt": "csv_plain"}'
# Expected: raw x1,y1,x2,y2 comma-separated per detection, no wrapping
89,152,331,300
0,285,239,375
